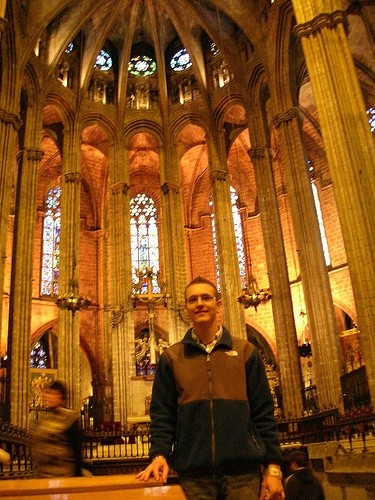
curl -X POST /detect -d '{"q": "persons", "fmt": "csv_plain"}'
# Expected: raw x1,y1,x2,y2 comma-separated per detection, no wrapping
136,336,149,367
136,274,287,500
157,336,170,355
31,380,84,478
33,372,51,407
281,450,326,500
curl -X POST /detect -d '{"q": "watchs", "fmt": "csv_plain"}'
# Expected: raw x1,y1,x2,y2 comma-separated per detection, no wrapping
263,467,284,478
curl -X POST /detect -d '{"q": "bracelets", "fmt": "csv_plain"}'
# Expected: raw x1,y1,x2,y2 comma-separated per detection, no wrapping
150,454,163,461
